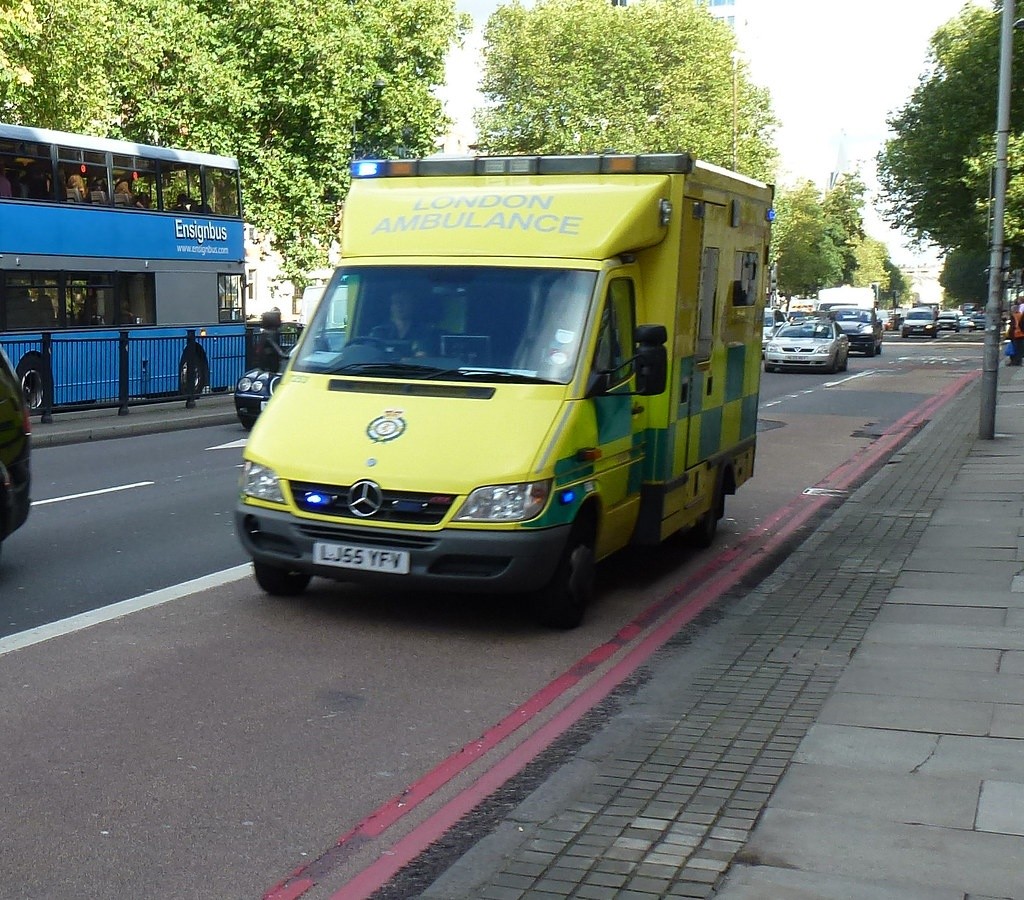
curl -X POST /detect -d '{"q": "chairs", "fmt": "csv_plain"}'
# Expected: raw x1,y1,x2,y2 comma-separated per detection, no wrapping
114,193,128,207
91,191,107,204
67,188,82,202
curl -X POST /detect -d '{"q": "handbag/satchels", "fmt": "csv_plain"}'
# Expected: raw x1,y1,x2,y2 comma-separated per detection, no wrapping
1004,342,1014,356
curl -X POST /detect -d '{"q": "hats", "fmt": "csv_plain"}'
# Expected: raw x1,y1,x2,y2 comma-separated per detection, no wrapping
1018,296,1024,303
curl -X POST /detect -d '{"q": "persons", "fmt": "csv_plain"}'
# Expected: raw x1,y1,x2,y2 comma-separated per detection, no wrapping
1007,299,1024,366
373,286,428,361
81,283,96,325
1,154,215,216
113,294,135,324
4,284,54,324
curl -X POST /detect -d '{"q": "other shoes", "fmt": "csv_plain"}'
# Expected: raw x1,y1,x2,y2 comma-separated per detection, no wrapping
1006,361,1022,366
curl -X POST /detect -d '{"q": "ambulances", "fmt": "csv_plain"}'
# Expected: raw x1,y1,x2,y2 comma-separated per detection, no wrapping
236,149,777,629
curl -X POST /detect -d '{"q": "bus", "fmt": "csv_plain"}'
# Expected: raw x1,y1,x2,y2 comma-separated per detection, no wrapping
0,120,250,420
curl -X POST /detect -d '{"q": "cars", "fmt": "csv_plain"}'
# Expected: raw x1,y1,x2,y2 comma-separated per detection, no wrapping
233,326,348,432
762,282,990,374
0,342,31,543
